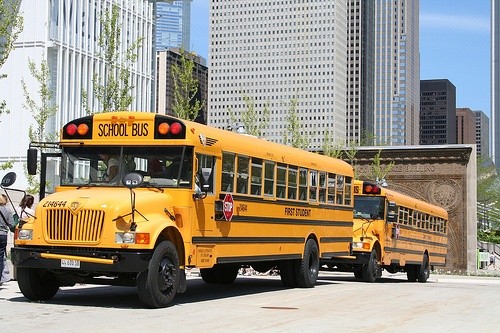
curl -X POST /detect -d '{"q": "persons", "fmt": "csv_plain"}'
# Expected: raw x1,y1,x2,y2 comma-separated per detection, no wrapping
97,146,128,183
161,157,191,182
0,194,16,289
17,195,35,218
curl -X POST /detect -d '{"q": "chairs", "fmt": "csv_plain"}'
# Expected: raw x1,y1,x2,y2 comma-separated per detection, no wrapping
225,177,305,201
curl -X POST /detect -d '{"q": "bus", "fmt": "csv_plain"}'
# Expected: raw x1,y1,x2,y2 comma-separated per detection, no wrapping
0,110,353,307
313,172,447,281
